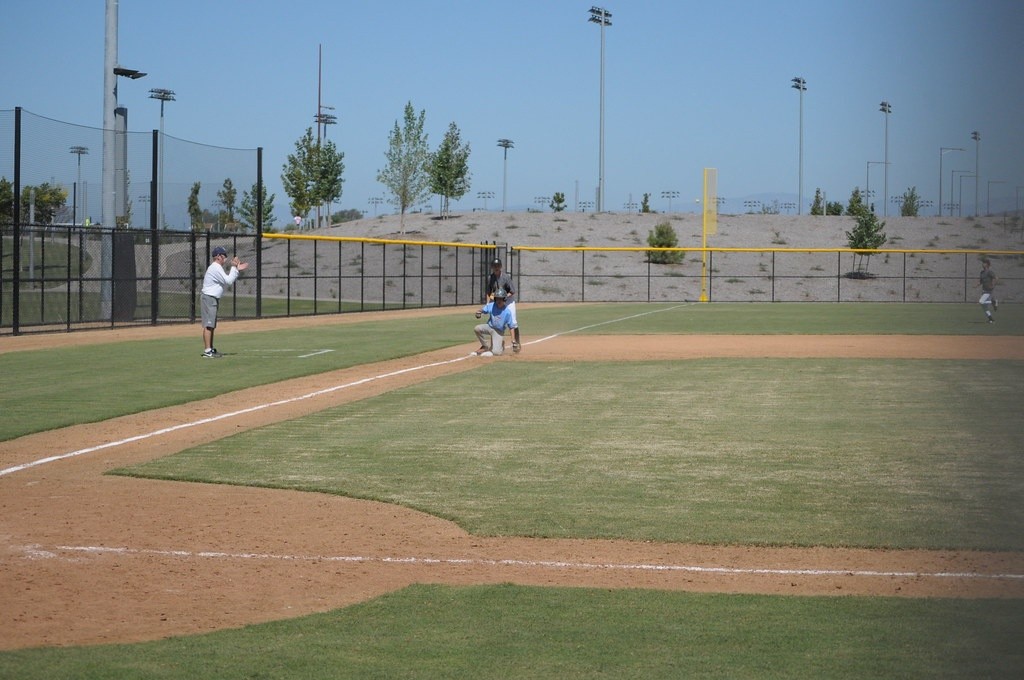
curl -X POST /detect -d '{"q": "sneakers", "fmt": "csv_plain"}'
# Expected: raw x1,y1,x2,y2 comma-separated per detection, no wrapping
203,348,221,358
502,341,505,351
476,345,489,353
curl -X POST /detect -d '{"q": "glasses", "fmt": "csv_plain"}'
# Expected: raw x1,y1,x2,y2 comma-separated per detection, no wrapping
221,254,227,258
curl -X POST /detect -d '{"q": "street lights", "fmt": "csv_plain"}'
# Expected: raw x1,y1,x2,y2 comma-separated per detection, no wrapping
780,202,797,213
860,189,877,209
790,73,809,215
367,197,385,217
476,191,497,210
147,86,177,230
579,201,595,213
68,145,90,225
496,138,516,212
969,130,981,218
533,196,551,212
660,190,681,213
958,174,977,216
742,200,761,212
137,194,152,229
587,5,616,212
879,100,892,218
950,170,976,215
937,147,967,217
623,202,639,213
211,200,224,231
866,160,895,210
986,179,1007,216
311,113,340,226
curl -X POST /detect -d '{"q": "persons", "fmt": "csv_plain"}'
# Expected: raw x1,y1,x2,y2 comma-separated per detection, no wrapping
974,257,999,325
85,216,90,228
871,202,875,215
470,258,522,357
293,213,302,230
200,245,249,360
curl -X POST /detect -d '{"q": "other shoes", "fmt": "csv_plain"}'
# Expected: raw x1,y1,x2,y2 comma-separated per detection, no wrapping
992,300,998,310
989,317,994,323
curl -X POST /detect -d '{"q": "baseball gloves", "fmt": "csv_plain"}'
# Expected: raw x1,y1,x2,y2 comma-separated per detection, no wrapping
490,292,494,300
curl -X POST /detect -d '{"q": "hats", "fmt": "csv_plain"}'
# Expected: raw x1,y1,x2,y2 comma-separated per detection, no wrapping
212,247,228,256
491,258,501,265
983,259,990,265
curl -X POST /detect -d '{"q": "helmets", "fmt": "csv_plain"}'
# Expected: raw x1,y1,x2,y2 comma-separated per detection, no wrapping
494,289,508,301
